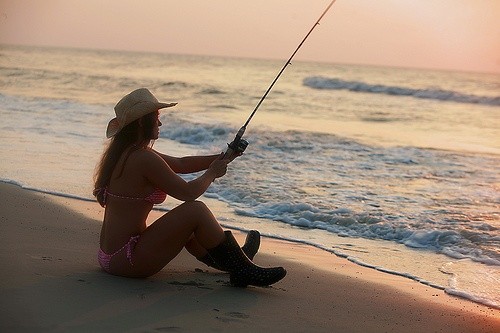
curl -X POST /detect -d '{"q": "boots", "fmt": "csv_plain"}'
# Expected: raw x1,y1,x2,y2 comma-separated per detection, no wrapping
206,229,287,286
197,229,260,271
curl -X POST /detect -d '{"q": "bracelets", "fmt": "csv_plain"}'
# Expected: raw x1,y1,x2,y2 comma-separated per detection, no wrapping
204,172,215,182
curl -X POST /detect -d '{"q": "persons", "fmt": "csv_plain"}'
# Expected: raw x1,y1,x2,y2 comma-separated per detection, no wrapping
92,89,287,289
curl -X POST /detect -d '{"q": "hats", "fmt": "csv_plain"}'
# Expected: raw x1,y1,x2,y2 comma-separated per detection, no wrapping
106,87,178,139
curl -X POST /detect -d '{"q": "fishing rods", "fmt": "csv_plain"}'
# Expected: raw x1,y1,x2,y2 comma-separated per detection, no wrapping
220,0,337,161
